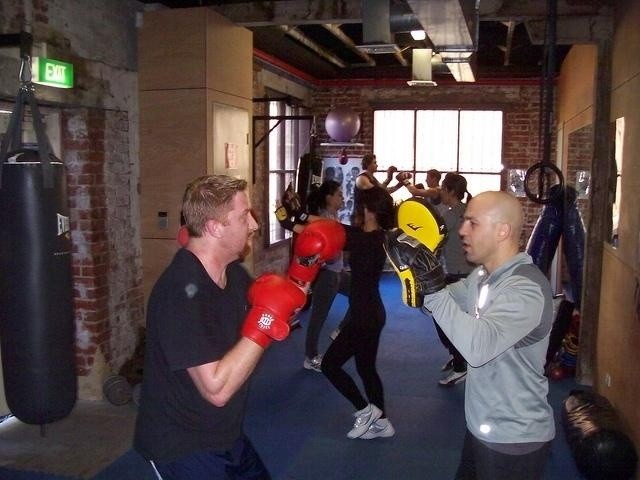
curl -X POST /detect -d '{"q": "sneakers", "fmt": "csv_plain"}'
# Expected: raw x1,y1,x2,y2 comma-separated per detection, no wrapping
438,371,467,387
303,354,323,373
358,420,395,439
441,355,454,372
330,328,340,341
347,404,382,439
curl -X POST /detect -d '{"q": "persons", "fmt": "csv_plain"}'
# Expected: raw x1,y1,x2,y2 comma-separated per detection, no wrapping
132,175,348,480
277,178,395,438
355,155,473,385
385,189,558,480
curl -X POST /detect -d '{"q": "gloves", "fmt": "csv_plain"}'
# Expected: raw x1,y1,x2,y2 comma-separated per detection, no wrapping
287,219,346,282
396,171,411,186
414,184,424,189
287,196,309,224
384,166,397,184
274,206,297,231
241,274,307,348
397,173,412,188
